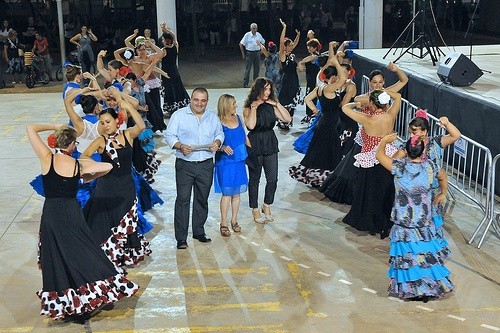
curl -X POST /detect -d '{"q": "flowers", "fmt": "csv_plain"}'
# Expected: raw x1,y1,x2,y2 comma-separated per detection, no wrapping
118,112,125,125
268,41,275,47
411,134,426,148
120,66,127,76
416,110,429,122
348,69,355,78
317,44,323,50
127,66,133,73
320,71,326,82
48,134,57,148
379,91,390,104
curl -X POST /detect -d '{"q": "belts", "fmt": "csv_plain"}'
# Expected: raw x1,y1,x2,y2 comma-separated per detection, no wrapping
246,50,260,52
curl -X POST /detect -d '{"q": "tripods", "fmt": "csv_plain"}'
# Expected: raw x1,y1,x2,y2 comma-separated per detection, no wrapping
384,0,446,67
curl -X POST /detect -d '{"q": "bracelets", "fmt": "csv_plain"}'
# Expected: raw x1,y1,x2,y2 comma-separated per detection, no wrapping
251,104,257,109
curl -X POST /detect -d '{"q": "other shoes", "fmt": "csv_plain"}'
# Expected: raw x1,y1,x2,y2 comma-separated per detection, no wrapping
177,240,188,249
278,125,290,130
12,81,22,84
380,228,389,239
192,234,211,243
244,84,248,88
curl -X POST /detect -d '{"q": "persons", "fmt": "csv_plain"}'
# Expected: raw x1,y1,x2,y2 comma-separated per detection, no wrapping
307,30,321,51
26,123,140,324
293,41,357,158
240,23,265,88
376,132,455,303
164,87,225,249
217,94,251,237
298,39,329,128
393,110,462,266
342,90,402,239
256,19,305,131
198,3,359,56
63,15,190,215
243,77,292,224
0,16,53,85
79,88,152,267
29,89,153,236
289,56,352,189
319,62,408,205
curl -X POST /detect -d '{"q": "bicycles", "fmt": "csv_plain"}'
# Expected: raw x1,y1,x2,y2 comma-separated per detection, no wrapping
24,50,96,88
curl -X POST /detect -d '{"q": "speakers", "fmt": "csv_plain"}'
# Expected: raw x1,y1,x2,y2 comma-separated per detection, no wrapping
436,53,484,87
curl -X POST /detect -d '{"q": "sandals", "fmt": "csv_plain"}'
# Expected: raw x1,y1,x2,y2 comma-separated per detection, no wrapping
220,223,231,237
231,219,241,232
252,208,267,224
261,203,274,222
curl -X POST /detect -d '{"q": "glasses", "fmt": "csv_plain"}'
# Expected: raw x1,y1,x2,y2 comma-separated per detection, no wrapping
74,140,79,145
